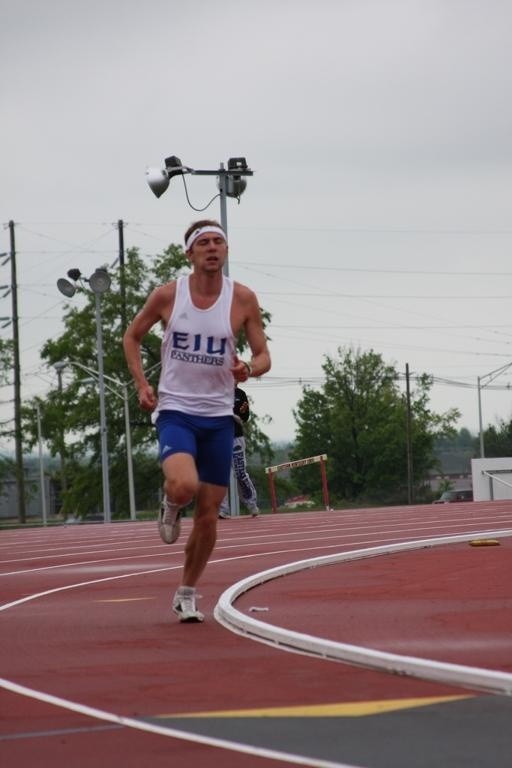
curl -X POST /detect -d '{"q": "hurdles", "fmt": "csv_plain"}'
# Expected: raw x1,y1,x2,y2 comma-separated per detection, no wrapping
264,454,329,512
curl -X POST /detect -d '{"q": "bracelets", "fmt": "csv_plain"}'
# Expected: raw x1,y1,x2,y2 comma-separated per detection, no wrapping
242,361,252,375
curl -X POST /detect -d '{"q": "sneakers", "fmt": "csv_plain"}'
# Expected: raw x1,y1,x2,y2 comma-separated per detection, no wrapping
158,494,182,544
219,512,230,519
171,590,204,622
251,507,258,517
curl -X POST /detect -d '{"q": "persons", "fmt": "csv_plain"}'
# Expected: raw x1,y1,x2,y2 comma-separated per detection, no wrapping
218,388,261,519
122,220,271,622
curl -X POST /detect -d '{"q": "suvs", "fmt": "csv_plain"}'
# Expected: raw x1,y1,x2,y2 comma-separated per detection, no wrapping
432,489,472,503
